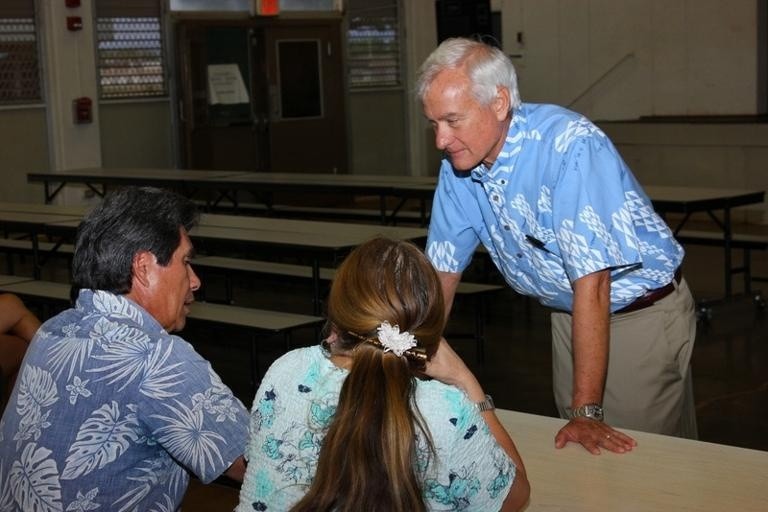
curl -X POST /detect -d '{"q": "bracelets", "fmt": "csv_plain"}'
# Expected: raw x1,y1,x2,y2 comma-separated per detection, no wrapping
473,394,497,413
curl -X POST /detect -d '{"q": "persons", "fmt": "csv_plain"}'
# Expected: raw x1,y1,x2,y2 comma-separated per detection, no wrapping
0,289,45,405
0,179,258,510
226,233,535,511
409,33,702,458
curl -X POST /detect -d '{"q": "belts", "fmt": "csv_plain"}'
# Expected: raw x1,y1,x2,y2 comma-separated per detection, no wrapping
613,265,681,314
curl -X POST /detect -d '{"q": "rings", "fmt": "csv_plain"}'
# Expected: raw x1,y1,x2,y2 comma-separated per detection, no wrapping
606,434,611,440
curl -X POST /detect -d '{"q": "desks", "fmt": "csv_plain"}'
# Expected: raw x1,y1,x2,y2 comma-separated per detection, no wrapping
27,168,768,314
493,409,768,512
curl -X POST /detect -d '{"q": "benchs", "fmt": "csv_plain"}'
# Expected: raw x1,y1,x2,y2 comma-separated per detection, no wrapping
0,198,768,387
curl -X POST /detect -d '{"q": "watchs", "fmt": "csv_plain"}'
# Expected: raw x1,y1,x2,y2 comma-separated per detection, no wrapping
570,403,606,422
